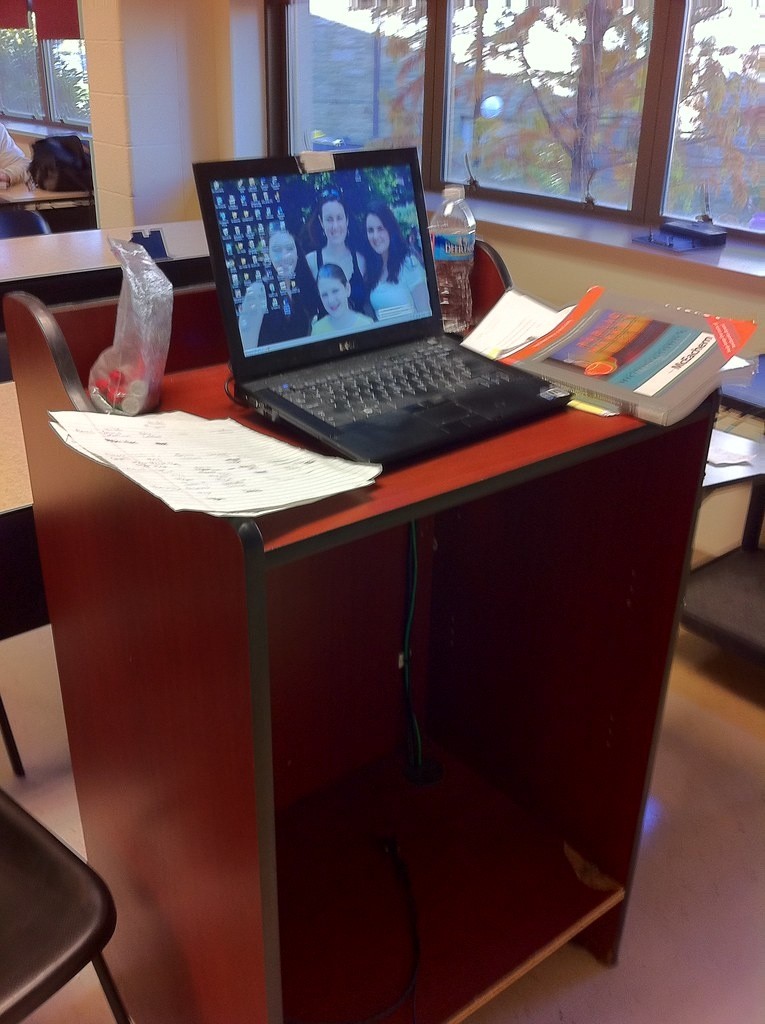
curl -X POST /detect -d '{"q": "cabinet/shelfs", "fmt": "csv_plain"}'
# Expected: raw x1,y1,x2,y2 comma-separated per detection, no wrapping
680,356,764,664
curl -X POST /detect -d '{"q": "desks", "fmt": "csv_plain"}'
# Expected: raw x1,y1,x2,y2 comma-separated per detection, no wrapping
0,180,95,216
0,220,210,331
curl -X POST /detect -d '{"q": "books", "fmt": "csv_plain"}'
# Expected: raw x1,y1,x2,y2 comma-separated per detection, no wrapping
499,285,758,426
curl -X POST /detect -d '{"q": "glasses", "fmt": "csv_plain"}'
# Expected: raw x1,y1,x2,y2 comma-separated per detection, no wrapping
320,189,339,199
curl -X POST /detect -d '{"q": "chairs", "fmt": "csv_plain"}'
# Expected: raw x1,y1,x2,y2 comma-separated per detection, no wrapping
0,788,131,1024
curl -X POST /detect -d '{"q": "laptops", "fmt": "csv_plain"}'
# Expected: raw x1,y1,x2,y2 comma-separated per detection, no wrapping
190,146,577,477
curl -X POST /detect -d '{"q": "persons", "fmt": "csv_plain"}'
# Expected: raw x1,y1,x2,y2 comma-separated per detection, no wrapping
0,121,32,190
238,190,431,349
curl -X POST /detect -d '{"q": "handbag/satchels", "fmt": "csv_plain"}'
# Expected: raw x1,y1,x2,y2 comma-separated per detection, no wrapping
29,136,94,193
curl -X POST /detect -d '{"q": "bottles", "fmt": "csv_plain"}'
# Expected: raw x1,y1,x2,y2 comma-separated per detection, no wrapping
429,185,478,333
